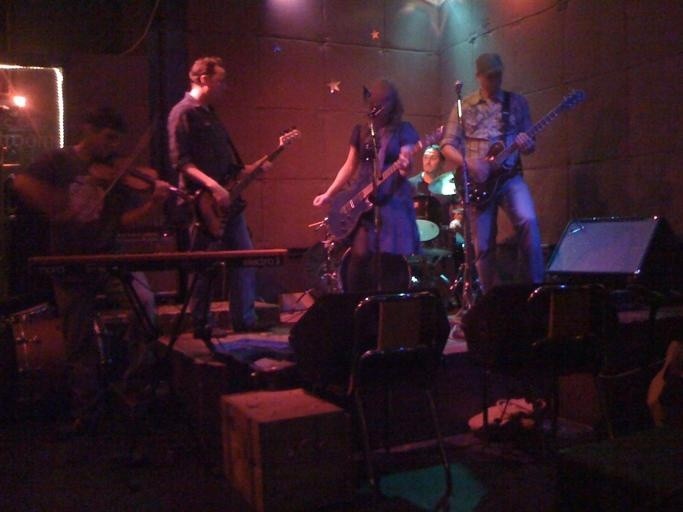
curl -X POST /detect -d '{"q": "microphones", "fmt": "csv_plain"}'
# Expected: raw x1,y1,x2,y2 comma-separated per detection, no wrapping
368,104,381,116
455,80,463,94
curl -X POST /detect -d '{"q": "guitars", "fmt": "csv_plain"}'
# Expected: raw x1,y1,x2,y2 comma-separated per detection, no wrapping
323,123,448,245
193,125,302,243
450,87,588,210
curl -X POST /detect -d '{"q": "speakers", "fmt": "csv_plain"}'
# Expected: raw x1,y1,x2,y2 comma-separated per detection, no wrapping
459,283,553,350
543,214,664,275
288,291,451,369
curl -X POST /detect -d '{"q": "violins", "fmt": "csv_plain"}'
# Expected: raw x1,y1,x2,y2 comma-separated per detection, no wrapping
87,160,199,201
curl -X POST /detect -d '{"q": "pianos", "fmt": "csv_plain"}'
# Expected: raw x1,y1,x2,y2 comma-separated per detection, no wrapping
25,247,290,277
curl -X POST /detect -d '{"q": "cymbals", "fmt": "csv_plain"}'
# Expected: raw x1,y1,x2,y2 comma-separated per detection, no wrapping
415,218,441,243
427,169,459,196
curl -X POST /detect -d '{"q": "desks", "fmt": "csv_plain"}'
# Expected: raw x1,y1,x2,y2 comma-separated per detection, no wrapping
28,248,291,337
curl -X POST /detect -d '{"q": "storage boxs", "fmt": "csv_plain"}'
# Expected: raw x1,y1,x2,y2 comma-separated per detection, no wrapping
222,389,345,512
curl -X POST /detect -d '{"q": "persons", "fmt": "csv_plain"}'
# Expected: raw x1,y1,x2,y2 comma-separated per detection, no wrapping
313,80,421,295
166,55,274,334
438,53,544,294
403,144,466,283
14,107,170,357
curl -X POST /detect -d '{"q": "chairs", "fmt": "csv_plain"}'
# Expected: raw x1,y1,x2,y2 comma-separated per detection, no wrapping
342,291,456,493
475,286,551,432
288,294,402,453
524,285,611,452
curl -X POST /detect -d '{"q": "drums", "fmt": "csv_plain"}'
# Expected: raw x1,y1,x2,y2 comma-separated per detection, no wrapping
318,241,413,302
410,192,443,224
1,311,108,426
